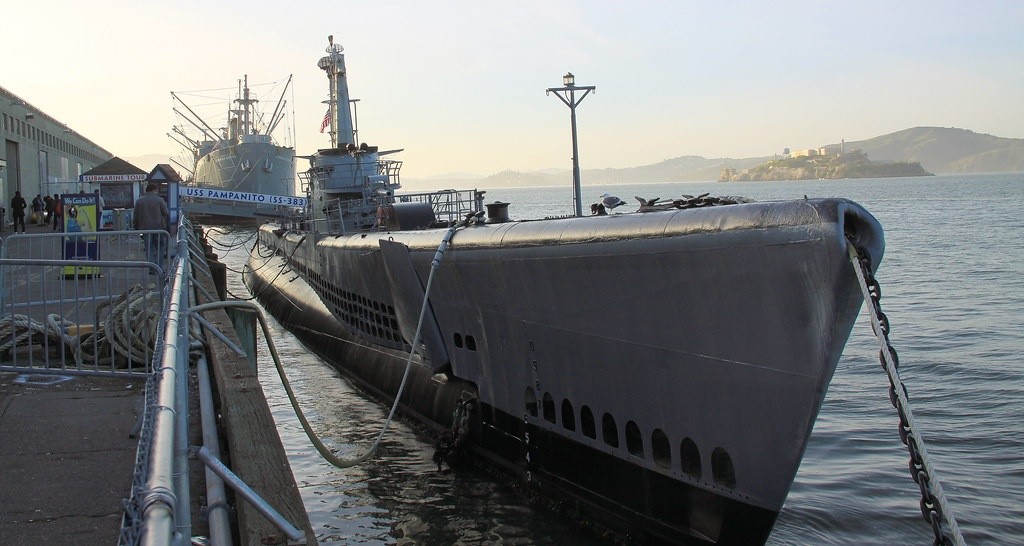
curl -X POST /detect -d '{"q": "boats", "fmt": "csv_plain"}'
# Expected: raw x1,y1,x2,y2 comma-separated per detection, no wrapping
243,35,885,546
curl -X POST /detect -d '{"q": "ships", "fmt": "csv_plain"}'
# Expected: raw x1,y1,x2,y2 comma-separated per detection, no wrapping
166,71,300,228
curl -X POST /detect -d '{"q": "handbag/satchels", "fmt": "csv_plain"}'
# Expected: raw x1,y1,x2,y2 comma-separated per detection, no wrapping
32,213,37,220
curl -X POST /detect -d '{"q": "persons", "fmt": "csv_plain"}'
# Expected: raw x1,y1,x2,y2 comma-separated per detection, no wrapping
94,189,104,229
11,191,26,235
32,193,62,232
132,182,169,275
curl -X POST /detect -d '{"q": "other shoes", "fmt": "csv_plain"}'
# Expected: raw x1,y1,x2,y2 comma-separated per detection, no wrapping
22,232,25,234
14,232,17,234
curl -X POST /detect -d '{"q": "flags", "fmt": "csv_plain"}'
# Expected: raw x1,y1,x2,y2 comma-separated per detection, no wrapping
320,110,331,133
